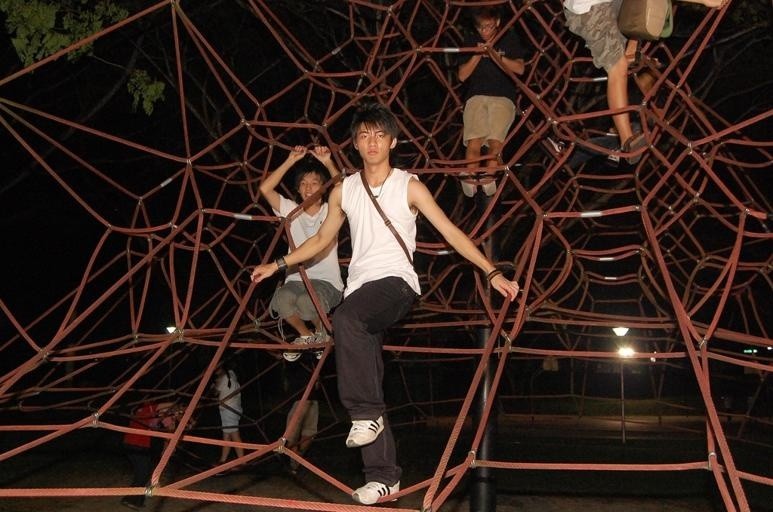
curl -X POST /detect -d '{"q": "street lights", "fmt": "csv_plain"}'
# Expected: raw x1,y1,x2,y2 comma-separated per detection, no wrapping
608,323,631,444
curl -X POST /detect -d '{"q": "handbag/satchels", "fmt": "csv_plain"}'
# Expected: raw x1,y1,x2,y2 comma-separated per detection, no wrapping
618,1,673,41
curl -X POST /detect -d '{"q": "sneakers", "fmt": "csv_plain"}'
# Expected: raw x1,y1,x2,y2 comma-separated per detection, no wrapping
459,172,497,198
345,416,384,448
352,480,400,505
283,332,330,361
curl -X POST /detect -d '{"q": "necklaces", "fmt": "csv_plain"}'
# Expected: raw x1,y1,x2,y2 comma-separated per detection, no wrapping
369,166,392,200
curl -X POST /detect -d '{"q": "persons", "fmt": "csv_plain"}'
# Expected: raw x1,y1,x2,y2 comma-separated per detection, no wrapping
250,103,520,505
260,145,344,361
282,349,320,477
212,363,246,477
120,402,175,510
563,0,729,165
457,6,525,198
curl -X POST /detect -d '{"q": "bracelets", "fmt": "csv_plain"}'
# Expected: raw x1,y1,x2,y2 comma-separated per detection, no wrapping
487,269,501,281
275,256,288,272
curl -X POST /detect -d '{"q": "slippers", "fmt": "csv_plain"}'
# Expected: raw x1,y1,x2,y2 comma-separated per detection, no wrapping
717,0,731,10
624,131,645,164
